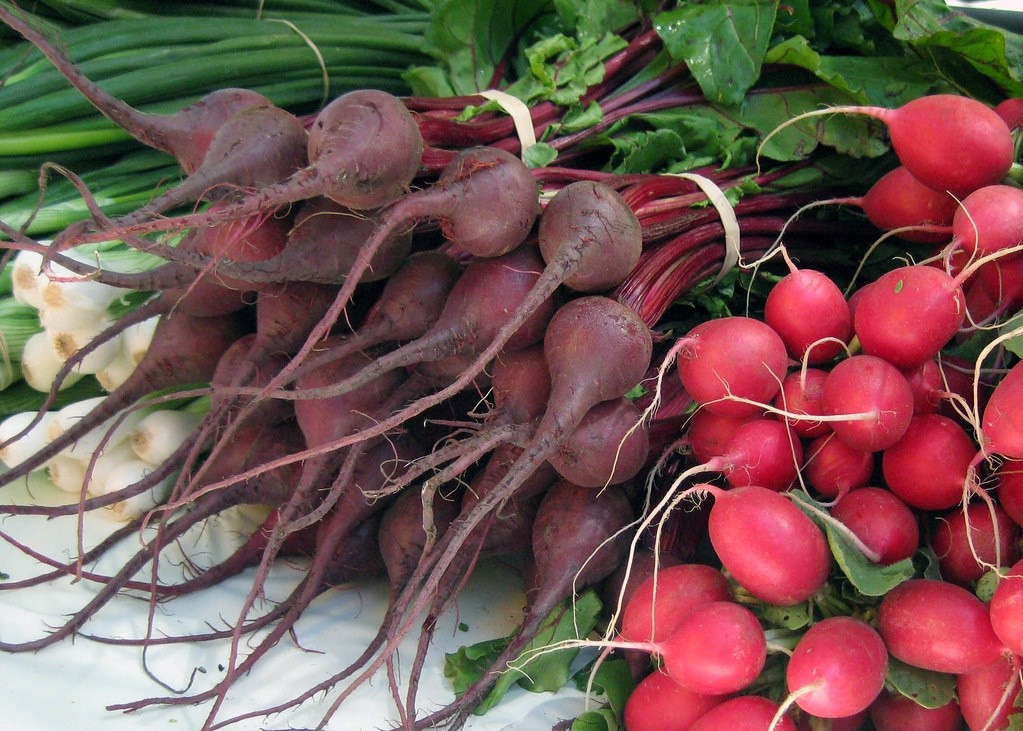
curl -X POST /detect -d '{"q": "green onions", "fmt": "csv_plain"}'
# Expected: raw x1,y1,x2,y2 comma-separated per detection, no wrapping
0,0,421,514
